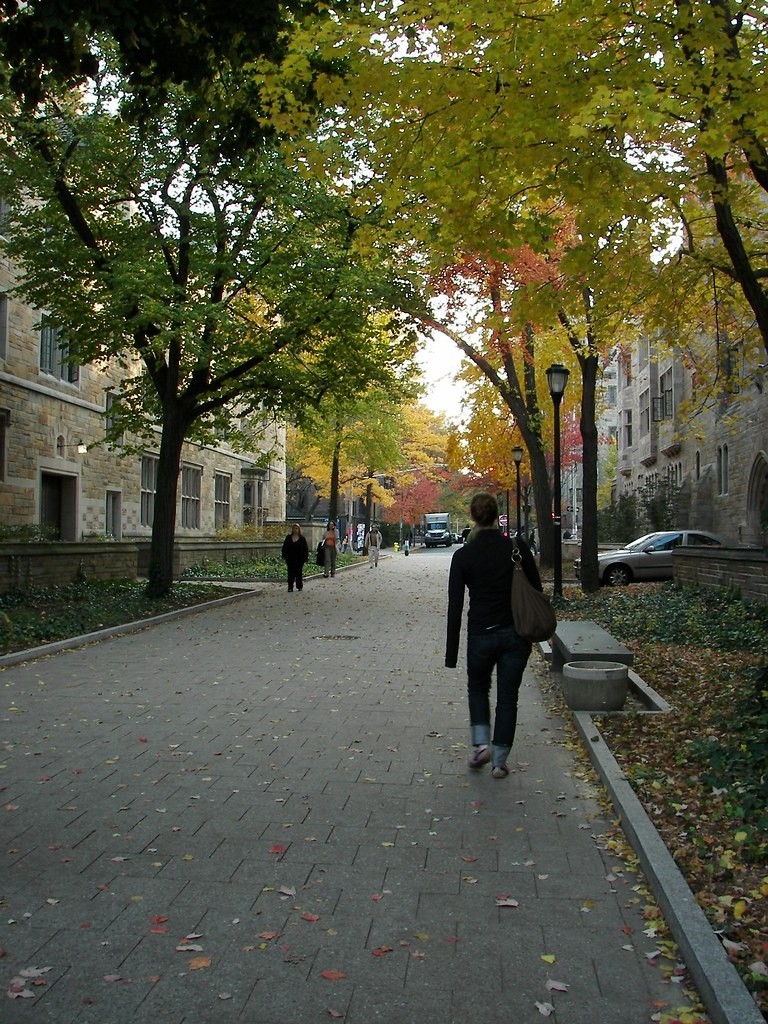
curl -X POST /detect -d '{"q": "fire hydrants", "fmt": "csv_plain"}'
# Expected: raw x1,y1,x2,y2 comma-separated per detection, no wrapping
394,542,400,552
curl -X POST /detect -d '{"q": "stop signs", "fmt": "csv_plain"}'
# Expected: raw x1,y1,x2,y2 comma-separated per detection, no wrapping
499,515,507,527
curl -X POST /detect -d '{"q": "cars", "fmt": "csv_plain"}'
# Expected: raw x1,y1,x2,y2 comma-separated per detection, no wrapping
572,530,757,587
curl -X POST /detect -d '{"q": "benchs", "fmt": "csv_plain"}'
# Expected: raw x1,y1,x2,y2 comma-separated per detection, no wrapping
549,620,634,672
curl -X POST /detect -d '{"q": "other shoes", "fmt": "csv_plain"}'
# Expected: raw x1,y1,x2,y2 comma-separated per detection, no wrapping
468,747,491,768
492,766,508,778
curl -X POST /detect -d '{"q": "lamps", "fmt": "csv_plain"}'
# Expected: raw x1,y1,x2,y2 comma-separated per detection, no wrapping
57,438,87,453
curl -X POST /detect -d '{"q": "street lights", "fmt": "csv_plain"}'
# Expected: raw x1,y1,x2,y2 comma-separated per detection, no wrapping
511,445,524,536
544,363,571,598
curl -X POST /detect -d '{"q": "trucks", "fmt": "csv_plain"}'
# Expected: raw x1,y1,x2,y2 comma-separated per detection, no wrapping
424,513,452,548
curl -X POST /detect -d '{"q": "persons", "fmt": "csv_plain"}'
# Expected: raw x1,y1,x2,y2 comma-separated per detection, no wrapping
364,524,382,568
445,493,542,778
322,520,339,578
462,524,471,542
282,523,309,593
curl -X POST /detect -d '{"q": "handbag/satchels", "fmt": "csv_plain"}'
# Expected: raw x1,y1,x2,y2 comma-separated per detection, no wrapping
316,539,325,566
511,536,557,643
